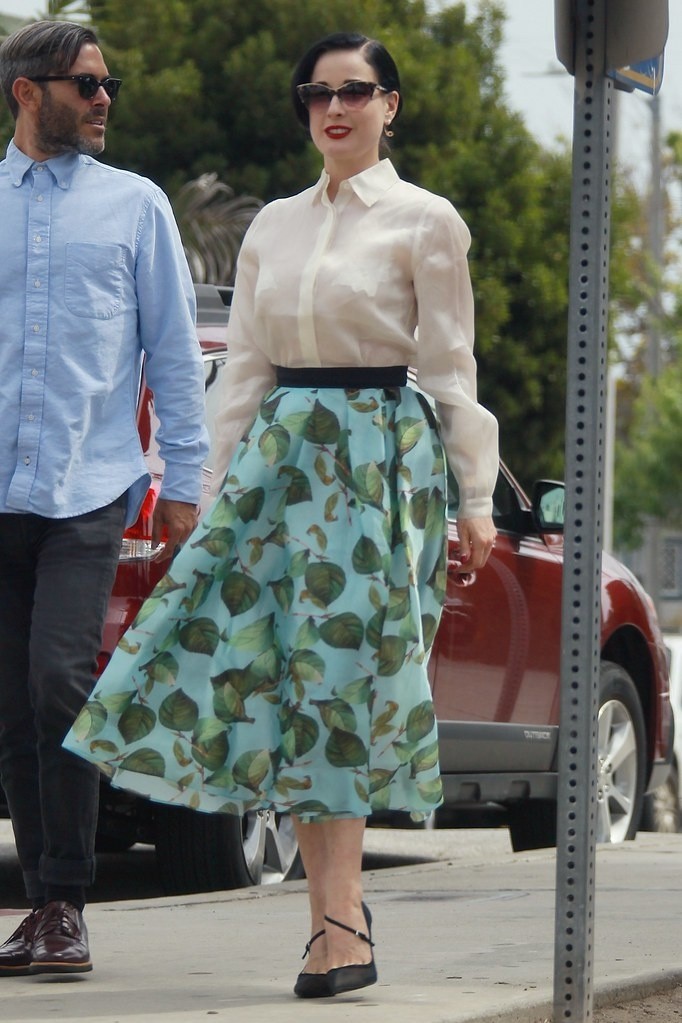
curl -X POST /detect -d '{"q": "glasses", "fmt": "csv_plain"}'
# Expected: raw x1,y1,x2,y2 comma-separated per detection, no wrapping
298,82,390,113
29,71,122,101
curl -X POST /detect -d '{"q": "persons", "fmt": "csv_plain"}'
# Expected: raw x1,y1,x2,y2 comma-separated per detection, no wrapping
0,21,208,976
58,31,500,996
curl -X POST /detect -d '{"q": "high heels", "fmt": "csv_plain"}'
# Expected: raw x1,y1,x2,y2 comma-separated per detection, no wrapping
292,928,333,999
0,911,41,974
325,902,378,996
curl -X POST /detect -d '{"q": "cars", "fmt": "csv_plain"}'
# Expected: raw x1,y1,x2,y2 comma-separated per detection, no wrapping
0,277,667,900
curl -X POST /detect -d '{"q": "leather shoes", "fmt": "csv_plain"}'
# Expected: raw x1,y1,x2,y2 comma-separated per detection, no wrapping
29,901,92,973
